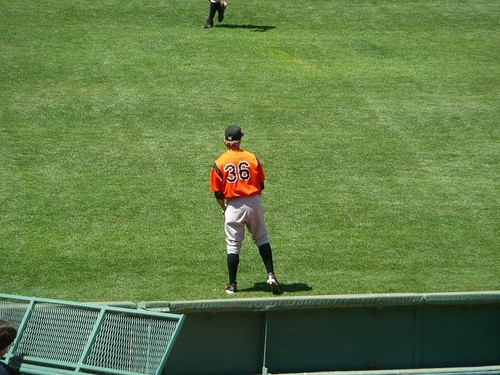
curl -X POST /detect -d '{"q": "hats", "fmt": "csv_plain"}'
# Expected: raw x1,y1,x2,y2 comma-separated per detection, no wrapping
225,126,244,141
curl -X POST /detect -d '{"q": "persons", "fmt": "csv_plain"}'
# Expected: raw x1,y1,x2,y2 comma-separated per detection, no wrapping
209,124,282,296
203,0,227,29
0,320,17,375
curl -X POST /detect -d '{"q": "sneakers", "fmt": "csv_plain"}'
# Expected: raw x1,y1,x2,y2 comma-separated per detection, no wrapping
218,2,227,22
267,273,281,295
202,20,213,28
224,283,236,293
